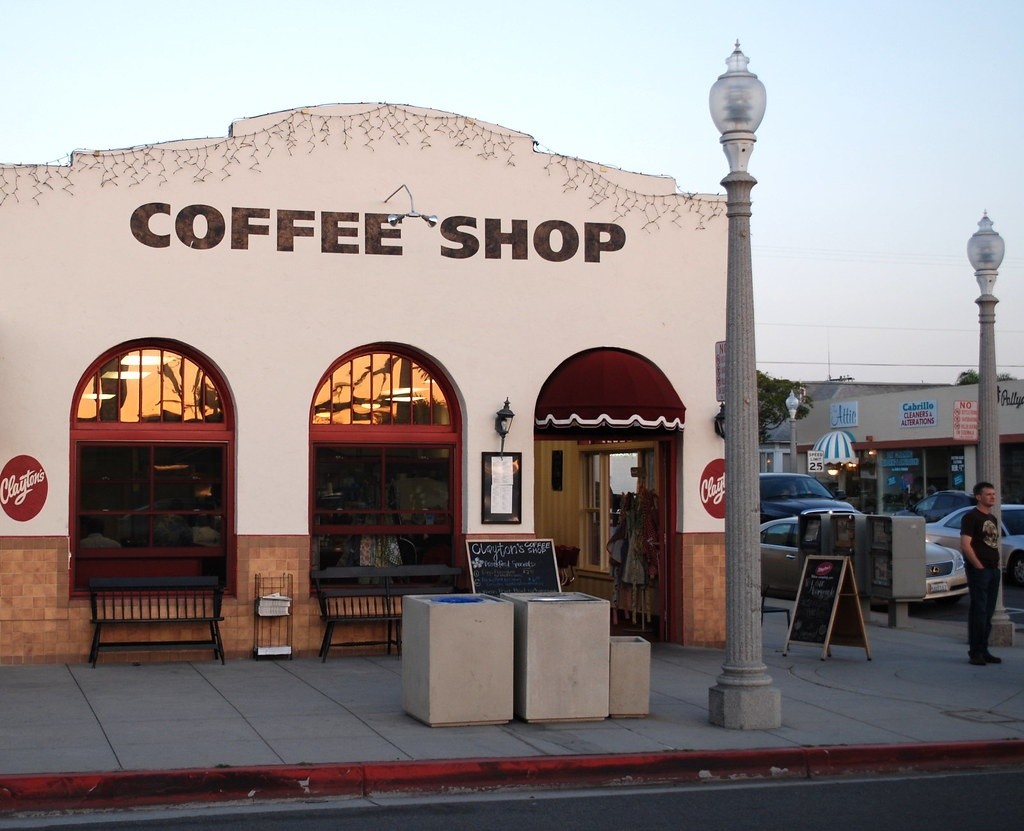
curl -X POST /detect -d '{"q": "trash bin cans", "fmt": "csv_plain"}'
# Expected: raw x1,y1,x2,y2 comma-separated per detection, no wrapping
798,514,926,629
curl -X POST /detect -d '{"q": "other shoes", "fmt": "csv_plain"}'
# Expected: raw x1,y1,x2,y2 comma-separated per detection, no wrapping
983,650,1002,664
968,657,986,665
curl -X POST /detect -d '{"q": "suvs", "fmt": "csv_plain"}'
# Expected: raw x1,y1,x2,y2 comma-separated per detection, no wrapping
759,472,855,526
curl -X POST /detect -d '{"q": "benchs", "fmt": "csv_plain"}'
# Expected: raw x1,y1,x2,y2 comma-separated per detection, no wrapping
311,564,474,663
86,575,226,670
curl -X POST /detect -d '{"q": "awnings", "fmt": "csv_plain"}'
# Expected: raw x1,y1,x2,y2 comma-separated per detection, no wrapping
534,349,686,434
812,431,859,466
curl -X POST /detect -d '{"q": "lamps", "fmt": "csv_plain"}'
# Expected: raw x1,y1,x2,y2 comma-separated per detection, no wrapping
714,401,727,441
497,397,515,452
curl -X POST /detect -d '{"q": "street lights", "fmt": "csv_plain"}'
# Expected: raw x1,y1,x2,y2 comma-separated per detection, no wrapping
964,213,1015,650
703,37,785,729
786,387,801,474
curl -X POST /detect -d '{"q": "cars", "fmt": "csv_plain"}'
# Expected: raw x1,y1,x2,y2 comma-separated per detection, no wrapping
890,489,977,524
925,504,1024,589
758,517,970,607
118,499,222,536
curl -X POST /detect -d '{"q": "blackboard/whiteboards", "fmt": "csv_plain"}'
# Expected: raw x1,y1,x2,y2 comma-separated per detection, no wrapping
466,539,562,598
787,555,870,648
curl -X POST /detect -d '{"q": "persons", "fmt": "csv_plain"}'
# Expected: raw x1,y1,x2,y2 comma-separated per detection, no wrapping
80,518,122,547
961,481,1004,665
785,481,806,495
